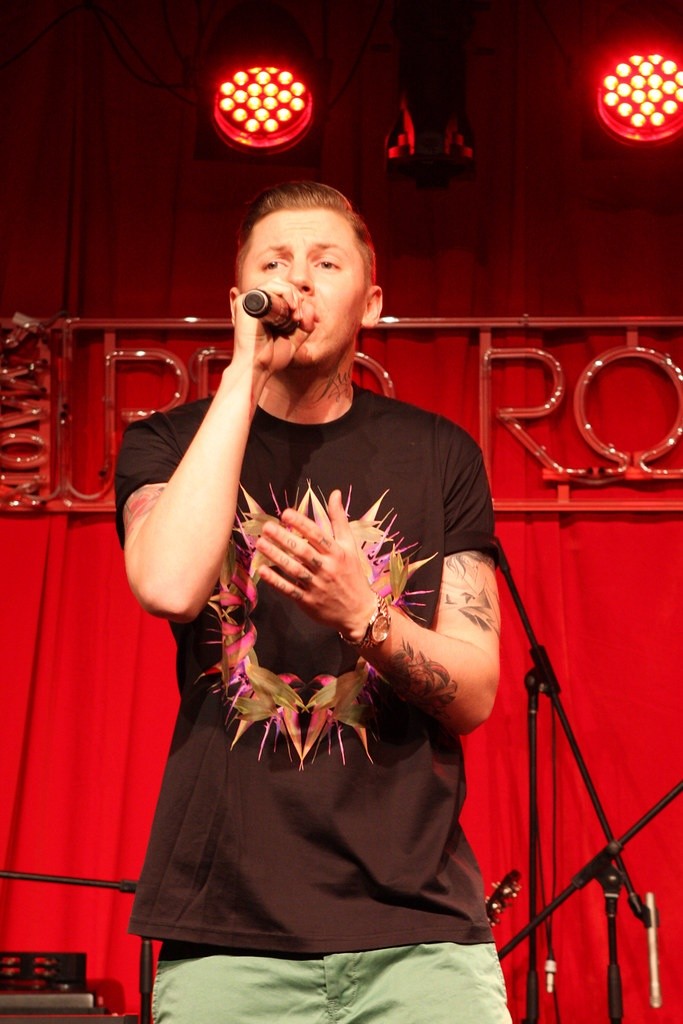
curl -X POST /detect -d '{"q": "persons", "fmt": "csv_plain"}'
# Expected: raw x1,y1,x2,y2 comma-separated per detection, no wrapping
114,180,511,1024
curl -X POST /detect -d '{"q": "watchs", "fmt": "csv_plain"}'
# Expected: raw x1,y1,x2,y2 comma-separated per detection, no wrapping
338,592,391,651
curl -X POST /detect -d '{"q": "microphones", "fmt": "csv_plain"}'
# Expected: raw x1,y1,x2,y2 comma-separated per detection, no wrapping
242,290,300,335
645,892,663,1009
